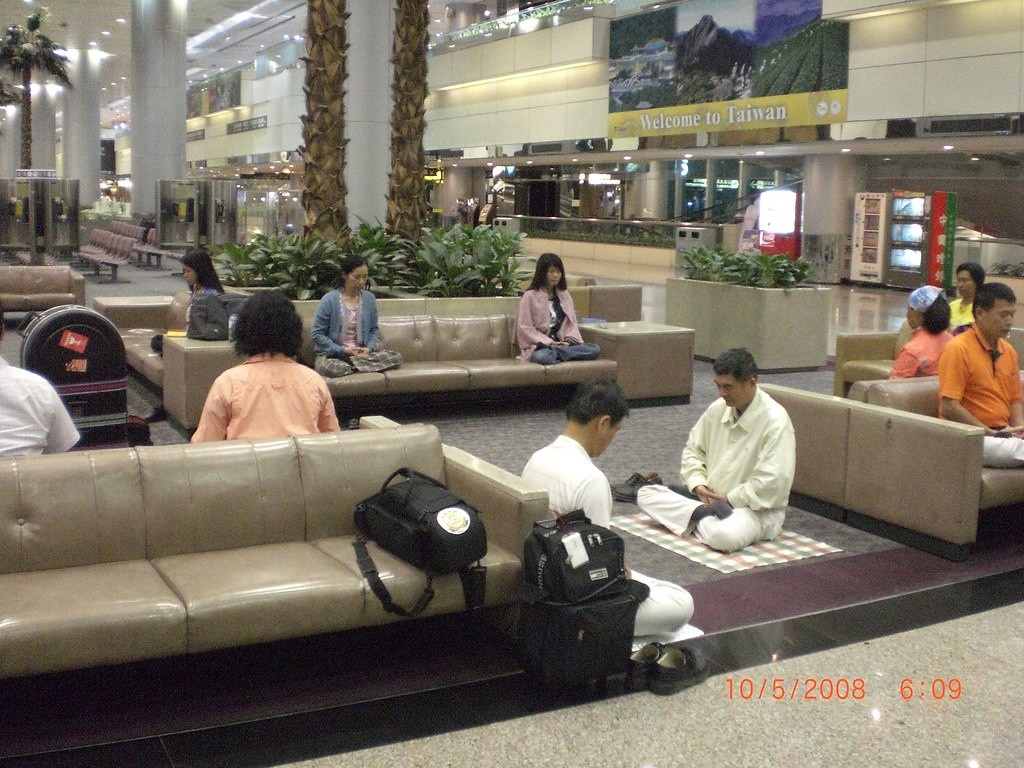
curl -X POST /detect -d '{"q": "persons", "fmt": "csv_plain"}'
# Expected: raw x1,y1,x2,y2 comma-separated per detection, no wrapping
637,349,796,550
889,285,954,379
151,250,225,355
311,256,403,377
0,298,81,457
512,253,601,364
523,379,695,636
939,282,1024,467
139,218,155,228
191,292,341,443
947,263,985,335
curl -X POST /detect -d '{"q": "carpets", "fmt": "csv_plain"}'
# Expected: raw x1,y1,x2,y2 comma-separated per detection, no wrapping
609,512,843,574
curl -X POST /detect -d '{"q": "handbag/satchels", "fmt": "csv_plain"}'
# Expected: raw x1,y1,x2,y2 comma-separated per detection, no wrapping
521,508,627,606
187,294,229,341
351,466,488,620
518,578,649,703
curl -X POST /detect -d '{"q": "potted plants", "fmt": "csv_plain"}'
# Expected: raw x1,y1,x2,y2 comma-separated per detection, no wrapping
662,246,835,375
201,220,536,342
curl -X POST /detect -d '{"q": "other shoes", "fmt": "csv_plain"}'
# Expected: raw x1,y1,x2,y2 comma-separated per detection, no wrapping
348,419,359,429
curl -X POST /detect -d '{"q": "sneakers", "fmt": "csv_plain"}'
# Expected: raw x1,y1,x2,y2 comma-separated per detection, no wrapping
626,642,673,692
649,647,711,695
609,472,663,502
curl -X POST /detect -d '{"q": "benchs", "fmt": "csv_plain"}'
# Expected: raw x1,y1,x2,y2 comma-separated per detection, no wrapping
756,322,1023,560
1,222,618,439
0,412,545,689
